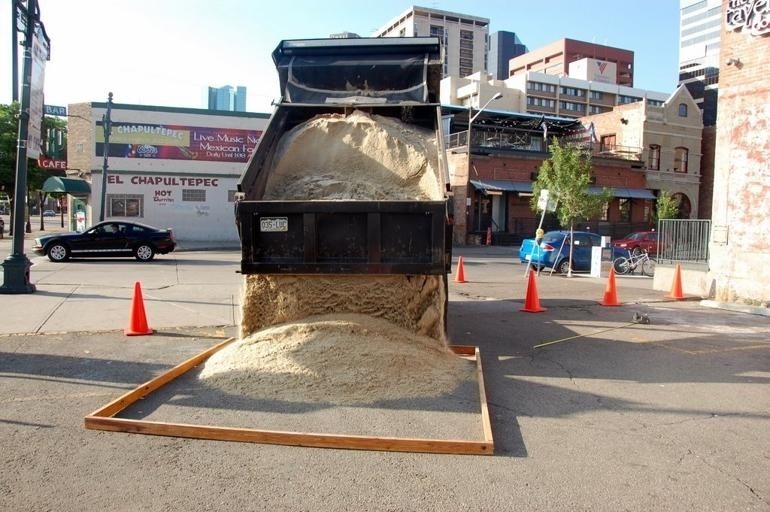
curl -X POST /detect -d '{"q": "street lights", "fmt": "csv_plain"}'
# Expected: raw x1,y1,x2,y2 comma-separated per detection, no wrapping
465,91,503,246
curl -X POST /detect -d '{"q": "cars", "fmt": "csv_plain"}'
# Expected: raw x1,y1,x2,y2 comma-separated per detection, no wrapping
517,230,629,273
42,209,56,217
611,231,674,255
31,219,177,262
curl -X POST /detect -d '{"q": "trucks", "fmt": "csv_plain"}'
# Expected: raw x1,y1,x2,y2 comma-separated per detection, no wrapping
233,37,454,276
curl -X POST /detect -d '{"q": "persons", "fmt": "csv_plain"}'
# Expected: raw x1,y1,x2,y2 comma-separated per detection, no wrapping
110,224,121,234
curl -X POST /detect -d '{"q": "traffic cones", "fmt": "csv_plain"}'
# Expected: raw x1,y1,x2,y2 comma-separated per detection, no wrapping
595,267,622,307
451,256,468,283
663,264,688,300
124,282,154,336
518,269,548,313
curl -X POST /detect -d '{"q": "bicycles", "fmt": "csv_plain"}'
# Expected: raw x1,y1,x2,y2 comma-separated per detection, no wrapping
613,242,658,277
